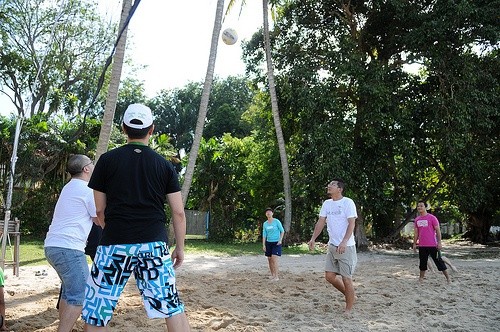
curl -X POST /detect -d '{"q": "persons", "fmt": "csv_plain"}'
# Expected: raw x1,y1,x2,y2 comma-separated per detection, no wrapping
0,267,10,332
308,179,358,314
427,233,458,274
55,222,100,309
81,104,192,332
413,201,451,283
263,208,284,281
44,155,101,332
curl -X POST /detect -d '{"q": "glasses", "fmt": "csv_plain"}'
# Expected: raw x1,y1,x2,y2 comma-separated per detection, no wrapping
82,160,94,171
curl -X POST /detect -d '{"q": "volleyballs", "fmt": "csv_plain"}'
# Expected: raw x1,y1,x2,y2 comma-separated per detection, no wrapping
222,28,238,45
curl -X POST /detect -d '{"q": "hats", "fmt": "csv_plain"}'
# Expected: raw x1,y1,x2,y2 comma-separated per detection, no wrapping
123,103,155,129
